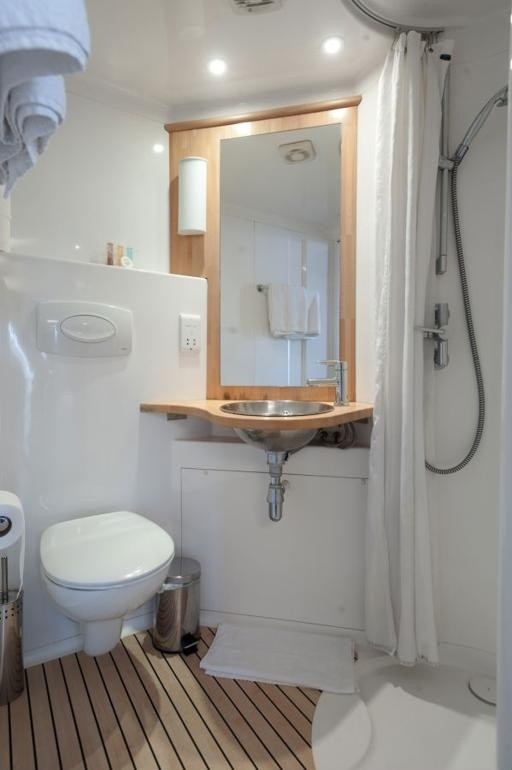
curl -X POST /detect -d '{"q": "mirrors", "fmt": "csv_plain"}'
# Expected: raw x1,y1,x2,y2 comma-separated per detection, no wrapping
220,121,342,389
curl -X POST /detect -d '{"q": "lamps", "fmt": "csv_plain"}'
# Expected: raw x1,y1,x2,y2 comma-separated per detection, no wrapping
177,154,208,240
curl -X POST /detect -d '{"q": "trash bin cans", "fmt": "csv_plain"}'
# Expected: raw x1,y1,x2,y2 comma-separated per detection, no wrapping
152,556,201,656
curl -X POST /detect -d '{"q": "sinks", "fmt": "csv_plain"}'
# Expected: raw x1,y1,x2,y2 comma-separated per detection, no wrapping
219,401,334,452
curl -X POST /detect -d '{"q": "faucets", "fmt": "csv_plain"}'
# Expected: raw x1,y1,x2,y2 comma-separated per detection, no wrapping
305,359,348,406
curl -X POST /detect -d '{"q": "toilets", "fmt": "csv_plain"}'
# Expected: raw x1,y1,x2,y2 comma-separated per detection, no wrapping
39,511,176,655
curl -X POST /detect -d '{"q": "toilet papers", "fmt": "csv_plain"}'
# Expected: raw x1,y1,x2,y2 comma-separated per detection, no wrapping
0,490,26,601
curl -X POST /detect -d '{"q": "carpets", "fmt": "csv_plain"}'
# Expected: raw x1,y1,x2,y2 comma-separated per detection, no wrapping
309,643,507,769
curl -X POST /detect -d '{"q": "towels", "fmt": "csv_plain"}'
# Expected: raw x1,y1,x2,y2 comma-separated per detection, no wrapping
261,285,326,340
0,1,93,198
197,620,366,700
